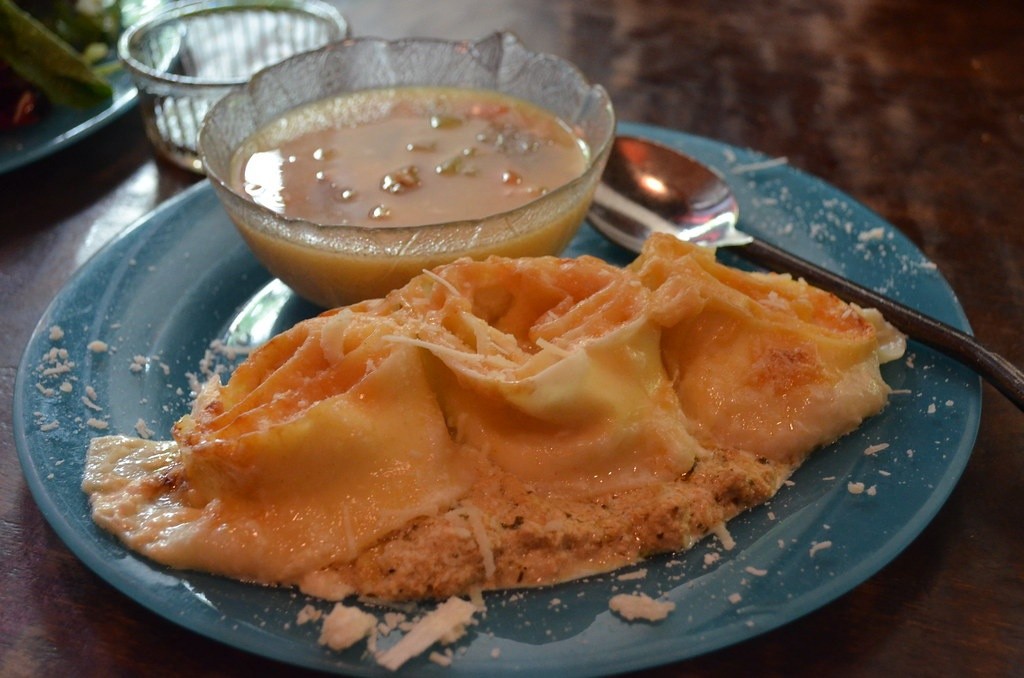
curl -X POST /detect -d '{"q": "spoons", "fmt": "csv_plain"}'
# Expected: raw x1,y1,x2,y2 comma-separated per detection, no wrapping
585,136,1024,410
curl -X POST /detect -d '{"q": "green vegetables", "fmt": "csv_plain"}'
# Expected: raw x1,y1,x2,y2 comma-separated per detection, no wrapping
0,0,133,146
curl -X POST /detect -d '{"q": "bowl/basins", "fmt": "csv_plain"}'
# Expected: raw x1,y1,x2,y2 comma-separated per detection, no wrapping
118,0,352,174
198,27,616,309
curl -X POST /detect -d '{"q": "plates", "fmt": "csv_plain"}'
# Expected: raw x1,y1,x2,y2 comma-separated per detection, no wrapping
10,122,979,678
0,0,185,174
